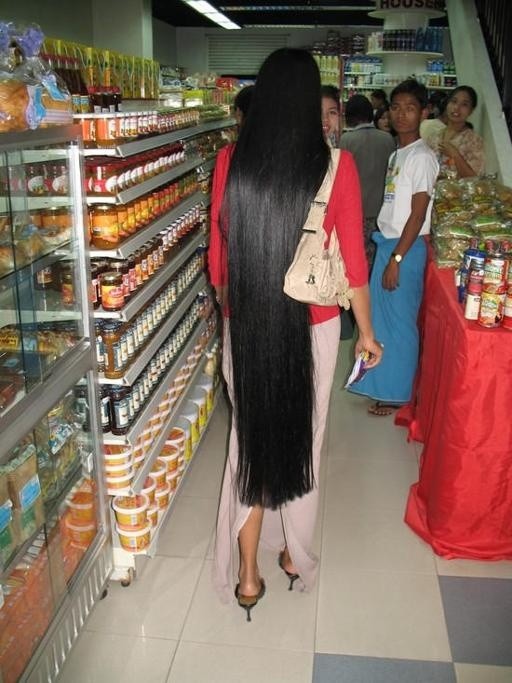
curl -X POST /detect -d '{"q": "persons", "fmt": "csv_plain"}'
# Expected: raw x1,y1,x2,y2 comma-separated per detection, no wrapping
233,85,254,131
204,46,385,605
321,80,489,416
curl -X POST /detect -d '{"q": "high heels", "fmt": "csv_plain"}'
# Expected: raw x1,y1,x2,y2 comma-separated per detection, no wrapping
234,577,265,622
278,548,300,591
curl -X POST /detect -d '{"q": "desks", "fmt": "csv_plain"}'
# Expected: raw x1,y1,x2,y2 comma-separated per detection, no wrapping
394,234,512,561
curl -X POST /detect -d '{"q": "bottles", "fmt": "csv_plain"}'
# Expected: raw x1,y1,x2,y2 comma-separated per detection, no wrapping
426,59,455,86
344,74,357,84
342,87,375,102
314,54,340,84
368,24,444,53
368,70,401,85
44,53,123,114
18,248,209,436
467,236,510,251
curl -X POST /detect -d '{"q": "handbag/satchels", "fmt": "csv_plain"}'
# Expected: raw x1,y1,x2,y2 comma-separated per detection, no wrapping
282,147,355,310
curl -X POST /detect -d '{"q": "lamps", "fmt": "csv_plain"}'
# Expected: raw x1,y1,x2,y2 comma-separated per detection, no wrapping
179,0,244,33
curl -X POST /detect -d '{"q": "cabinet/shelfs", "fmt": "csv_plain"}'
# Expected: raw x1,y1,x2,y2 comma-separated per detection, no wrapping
310,44,459,117
22,115,240,583
0,119,117,681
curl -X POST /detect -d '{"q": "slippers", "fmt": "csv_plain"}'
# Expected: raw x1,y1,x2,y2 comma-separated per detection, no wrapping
367,400,399,415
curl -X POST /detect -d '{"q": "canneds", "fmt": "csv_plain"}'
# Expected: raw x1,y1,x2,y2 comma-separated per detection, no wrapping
479,252,512,332
0,91,205,435
